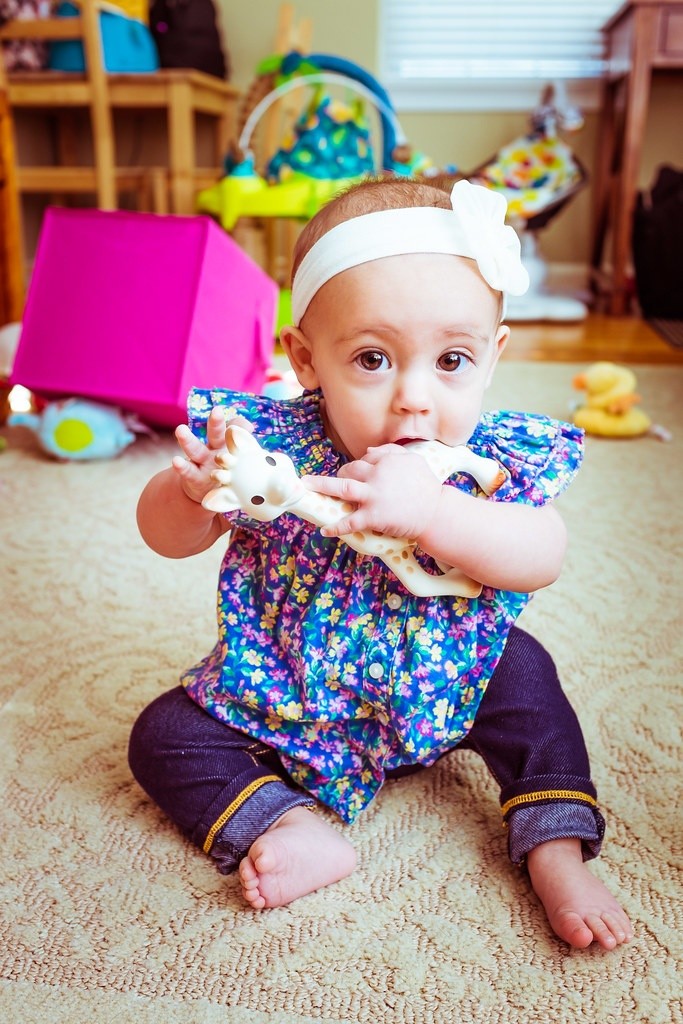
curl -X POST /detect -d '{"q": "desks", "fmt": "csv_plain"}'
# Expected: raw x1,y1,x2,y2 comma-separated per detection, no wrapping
579,1,682,315
0,68,247,233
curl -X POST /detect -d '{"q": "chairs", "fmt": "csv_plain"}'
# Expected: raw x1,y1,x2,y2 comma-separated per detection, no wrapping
0,2,171,326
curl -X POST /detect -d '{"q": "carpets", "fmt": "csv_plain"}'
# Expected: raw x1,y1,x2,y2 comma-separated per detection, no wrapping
0,355,682,1024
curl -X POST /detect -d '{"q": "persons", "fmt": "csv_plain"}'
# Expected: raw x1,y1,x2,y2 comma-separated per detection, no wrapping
125,176,635,953
